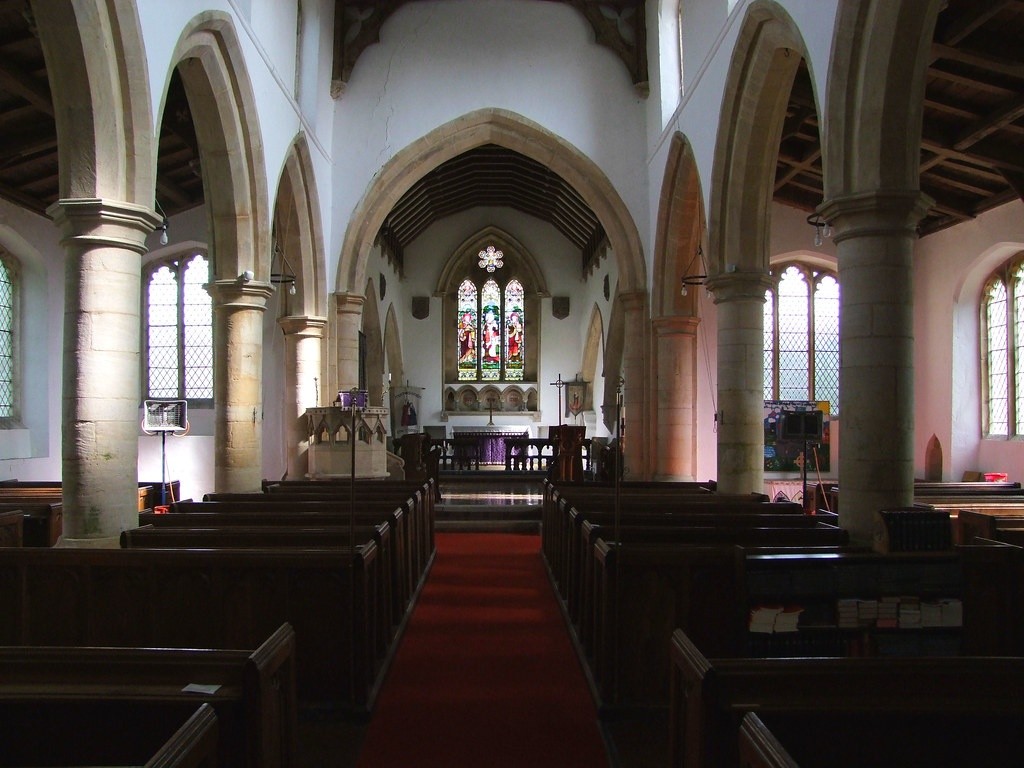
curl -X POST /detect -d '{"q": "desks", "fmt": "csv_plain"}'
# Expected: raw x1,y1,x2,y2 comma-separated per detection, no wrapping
452,429,529,466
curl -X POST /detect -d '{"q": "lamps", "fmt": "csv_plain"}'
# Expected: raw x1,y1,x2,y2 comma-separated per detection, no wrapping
142,397,190,507
262,218,301,296
804,198,837,247
777,404,826,514
153,200,175,247
678,182,718,298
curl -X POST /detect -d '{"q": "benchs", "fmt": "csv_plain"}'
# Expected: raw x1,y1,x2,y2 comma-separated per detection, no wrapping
0,476,1024,768
411,435,592,472
